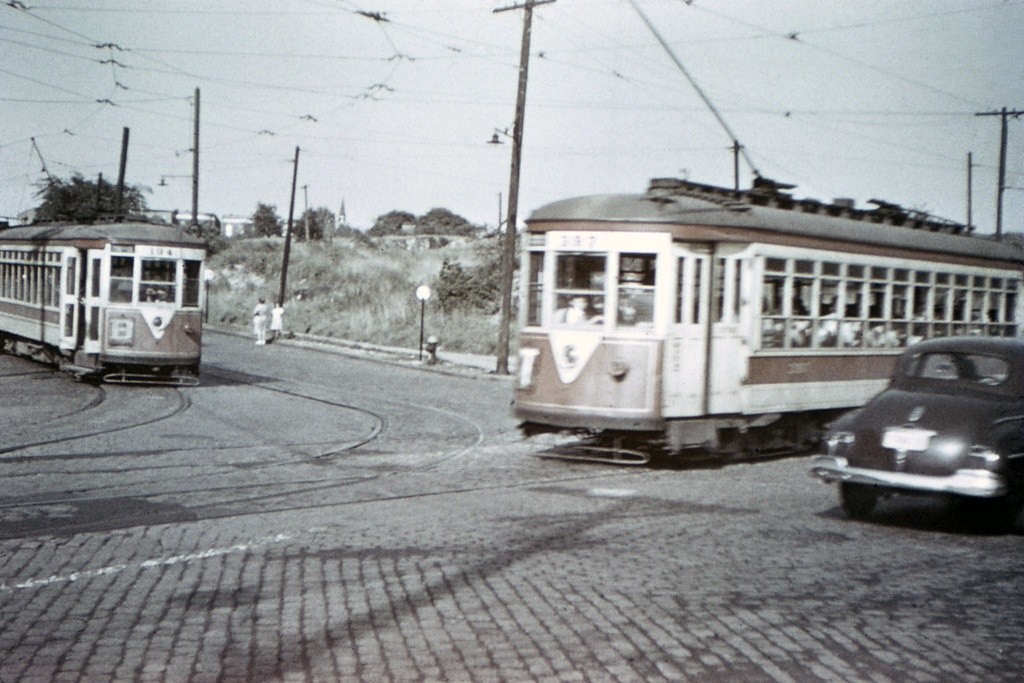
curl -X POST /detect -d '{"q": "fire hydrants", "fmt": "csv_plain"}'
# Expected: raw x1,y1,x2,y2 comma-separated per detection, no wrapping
423,335,441,365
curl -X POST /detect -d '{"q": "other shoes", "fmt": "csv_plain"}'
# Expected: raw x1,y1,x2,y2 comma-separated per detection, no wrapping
255,339,266,345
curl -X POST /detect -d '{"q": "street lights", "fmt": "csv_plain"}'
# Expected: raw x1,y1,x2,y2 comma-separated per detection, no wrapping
487,128,524,376
416,280,433,359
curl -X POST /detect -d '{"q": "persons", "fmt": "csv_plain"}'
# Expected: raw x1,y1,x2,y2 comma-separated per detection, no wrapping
254,297,267,345
270,302,285,337
555,295,604,324
146,274,166,303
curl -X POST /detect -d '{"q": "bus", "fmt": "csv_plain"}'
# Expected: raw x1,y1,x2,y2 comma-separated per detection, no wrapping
0,205,215,386
511,176,1024,465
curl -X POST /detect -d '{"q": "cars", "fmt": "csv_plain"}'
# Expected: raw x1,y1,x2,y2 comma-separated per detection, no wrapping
809,336,1024,526
